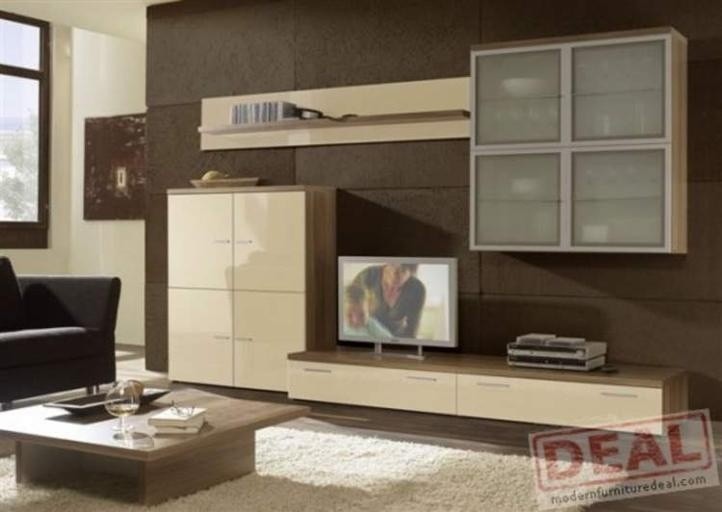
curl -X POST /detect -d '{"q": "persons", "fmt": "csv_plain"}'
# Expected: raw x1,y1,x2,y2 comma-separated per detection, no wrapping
352,263,426,340
343,284,390,339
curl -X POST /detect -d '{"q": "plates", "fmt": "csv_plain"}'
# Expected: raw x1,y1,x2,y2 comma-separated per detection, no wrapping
42,384,171,415
190,176,259,189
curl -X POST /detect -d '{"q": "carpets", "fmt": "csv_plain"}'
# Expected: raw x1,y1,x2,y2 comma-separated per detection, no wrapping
0,415,630,512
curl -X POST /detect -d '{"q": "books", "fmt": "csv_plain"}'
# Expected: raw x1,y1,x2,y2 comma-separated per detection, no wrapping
154,417,206,435
147,405,209,426
228,101,297,123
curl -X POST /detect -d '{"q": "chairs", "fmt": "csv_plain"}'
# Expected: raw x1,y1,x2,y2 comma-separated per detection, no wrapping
0,255,123,409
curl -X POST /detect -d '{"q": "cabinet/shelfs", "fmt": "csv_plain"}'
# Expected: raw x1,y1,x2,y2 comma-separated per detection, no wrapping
166,24,688,435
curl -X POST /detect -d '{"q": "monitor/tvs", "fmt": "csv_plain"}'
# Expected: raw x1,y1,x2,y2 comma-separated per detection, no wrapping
336,256,460,360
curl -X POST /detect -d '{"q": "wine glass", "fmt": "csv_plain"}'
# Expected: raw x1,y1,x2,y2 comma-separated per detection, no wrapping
105,379,141,432
112,430,155,450
